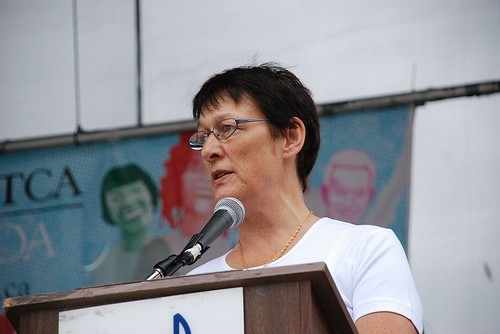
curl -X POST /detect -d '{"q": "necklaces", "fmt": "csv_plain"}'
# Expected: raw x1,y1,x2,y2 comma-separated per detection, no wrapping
237,210,315,271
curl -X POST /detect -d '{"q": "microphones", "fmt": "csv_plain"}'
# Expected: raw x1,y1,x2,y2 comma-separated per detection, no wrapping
181,197,246,266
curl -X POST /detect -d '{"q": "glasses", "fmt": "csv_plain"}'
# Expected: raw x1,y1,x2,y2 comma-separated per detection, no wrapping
188,118,288,151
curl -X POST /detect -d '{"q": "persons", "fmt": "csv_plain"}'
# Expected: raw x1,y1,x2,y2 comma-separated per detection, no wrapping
182,62,424,334
